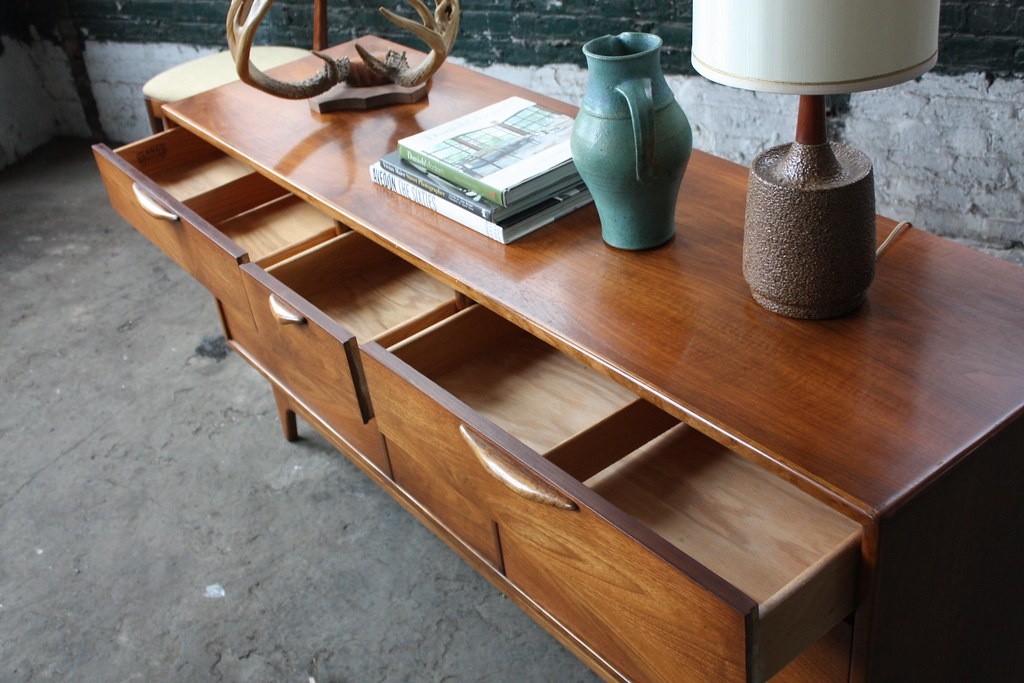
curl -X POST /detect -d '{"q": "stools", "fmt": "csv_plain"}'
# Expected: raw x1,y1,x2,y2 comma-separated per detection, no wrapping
142,45,312,135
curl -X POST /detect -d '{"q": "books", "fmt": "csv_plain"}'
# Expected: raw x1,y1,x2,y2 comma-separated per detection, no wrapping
368,96,597,244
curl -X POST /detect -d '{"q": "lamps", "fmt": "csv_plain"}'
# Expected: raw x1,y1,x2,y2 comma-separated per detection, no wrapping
689,0,940,320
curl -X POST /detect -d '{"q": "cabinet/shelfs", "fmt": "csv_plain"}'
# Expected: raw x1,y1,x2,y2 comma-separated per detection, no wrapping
92,34,1024,683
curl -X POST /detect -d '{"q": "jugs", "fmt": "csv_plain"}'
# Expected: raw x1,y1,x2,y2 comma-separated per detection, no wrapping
570,32,693,249
